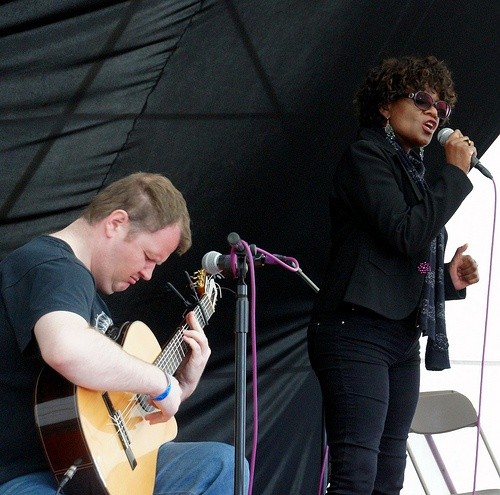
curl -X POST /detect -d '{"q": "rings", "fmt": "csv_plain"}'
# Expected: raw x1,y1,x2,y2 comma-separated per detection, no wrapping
465,139,471,147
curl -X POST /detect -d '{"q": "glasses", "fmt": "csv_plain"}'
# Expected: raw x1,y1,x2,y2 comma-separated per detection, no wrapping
397,91,451,120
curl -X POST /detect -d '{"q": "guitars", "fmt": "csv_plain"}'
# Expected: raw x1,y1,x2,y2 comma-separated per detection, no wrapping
33,263,223,495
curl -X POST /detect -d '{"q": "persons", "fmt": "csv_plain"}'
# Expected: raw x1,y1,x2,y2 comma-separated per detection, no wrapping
0,172,250,495
307,54,479,495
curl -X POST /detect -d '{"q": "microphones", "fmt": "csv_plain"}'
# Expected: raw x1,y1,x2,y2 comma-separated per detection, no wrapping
201,250,289,275
437,128,493,181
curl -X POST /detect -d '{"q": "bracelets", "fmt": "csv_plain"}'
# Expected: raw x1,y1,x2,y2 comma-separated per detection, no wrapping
145,368,171,402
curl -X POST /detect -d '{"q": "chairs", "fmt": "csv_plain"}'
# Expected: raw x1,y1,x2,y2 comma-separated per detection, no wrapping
405,389,500,494
